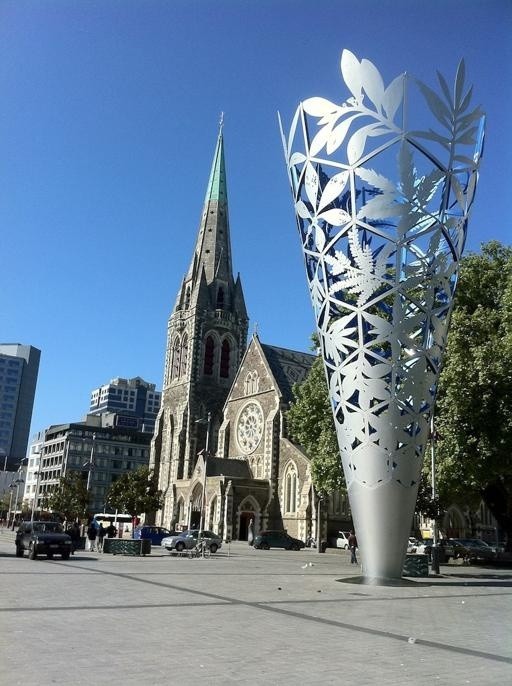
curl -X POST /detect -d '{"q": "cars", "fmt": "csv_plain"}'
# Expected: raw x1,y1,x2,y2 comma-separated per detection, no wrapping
133,526,175,548
327,530,356,551
253,530,306,552
161,529,222,554
406,533,498,558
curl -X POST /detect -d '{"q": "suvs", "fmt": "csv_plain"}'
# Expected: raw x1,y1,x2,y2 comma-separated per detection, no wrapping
16,522,72,560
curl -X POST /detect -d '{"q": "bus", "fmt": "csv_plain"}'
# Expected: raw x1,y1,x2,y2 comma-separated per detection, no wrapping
93,514,141,534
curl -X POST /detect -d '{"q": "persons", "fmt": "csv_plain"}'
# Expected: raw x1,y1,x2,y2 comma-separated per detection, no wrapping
87,522,97,551
97,523,106,548
348,529,358,564
72,523,80,555
106,520,116,539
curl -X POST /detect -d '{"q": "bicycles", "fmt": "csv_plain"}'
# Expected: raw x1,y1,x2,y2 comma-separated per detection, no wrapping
188,536,211,559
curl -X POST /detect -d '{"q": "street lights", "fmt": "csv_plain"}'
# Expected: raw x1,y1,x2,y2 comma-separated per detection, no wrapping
195,411,212,541
11,466,26,531
316,489,327,553
31,447,45,522
81,432,99,526
6,476,18,529
